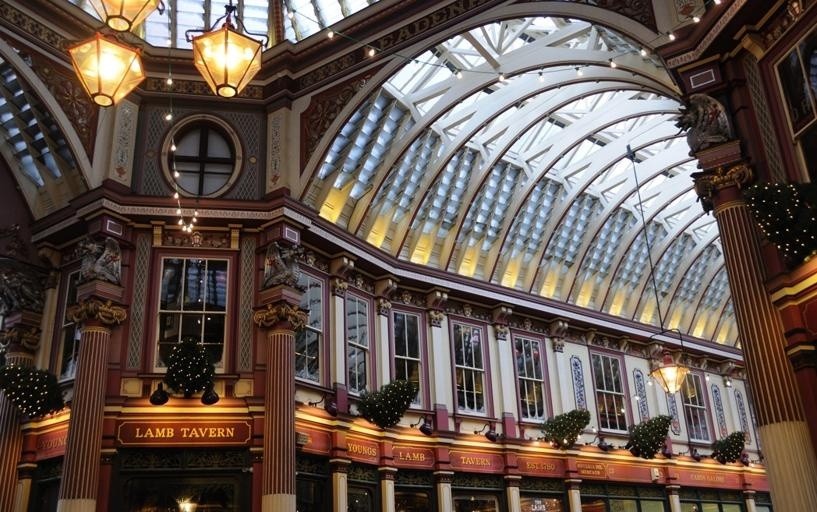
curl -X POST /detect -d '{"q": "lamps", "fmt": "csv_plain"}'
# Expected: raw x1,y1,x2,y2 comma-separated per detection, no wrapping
473,423,496,442
148,378,219,407
586,434,748,467
410,413,432,436
68,0,269,111
624,147,691,397
307,390,337,417
